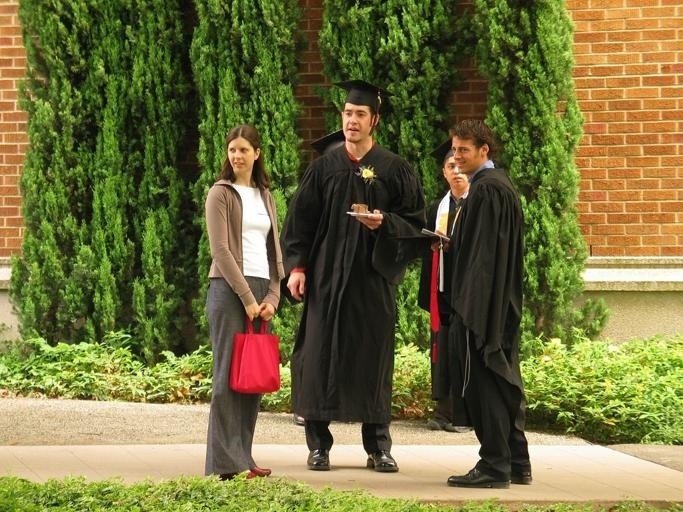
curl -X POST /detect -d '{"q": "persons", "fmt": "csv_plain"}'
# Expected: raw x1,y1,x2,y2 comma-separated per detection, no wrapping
280,79,428,472
417,149,476,432
294,129,347,425
206,125,285,480
431,120,533,488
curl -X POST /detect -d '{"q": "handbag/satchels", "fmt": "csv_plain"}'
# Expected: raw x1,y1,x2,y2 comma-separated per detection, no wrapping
229,307,282,394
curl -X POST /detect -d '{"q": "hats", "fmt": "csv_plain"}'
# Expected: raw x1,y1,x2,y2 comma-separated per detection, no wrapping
333,79,396,114
428,136,456,163
311,126,345,156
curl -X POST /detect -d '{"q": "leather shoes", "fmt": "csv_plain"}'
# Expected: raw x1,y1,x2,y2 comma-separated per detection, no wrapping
293,410,305,425
510,459,534,483
306,447,332,470
365,450,399,473
220,464,272,480
447,458,512,488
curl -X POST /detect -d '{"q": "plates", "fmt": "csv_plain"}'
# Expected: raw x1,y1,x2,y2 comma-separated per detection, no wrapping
346,211,375,218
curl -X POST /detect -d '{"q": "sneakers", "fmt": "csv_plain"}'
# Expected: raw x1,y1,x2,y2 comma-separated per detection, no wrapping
426,410,474,434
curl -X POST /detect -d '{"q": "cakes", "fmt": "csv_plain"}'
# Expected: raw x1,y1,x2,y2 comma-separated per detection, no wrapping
352,204,368,214
